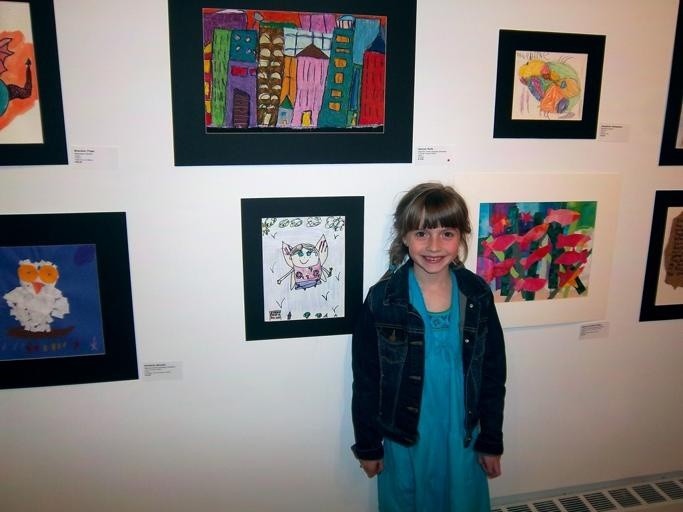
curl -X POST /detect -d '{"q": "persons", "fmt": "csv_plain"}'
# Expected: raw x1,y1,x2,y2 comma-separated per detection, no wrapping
352,183,507,511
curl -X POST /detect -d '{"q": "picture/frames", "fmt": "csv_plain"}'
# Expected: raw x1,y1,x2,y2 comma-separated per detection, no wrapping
166,0,417,166
654,0,680,166
0,0,69,167
636,188,681,322
491,27,606,140
240,195,365,342
453,172,621,330
0,209,139,391
